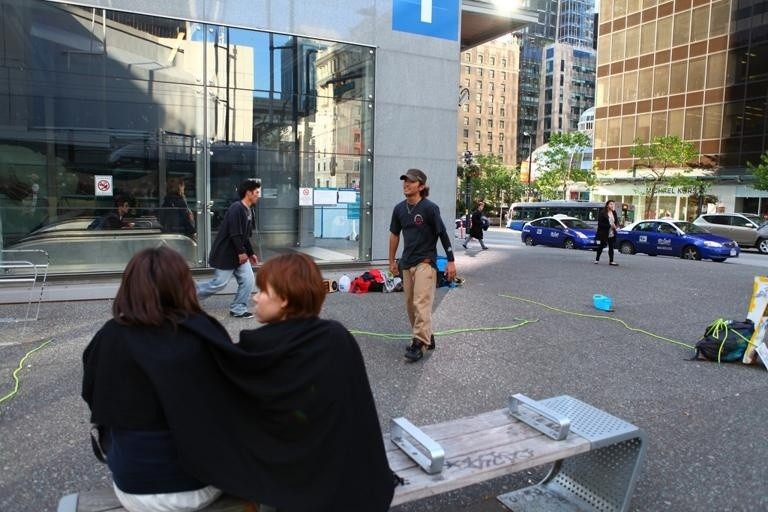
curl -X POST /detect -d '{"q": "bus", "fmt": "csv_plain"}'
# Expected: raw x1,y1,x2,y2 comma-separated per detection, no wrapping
95,140,280,217
506,201,636,237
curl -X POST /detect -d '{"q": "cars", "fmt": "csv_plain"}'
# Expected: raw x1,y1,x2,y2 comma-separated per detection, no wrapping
522,212,606,251
613,219,739,262
456,213,489,232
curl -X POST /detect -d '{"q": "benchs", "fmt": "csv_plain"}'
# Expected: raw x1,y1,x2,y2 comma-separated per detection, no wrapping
57,391,649,510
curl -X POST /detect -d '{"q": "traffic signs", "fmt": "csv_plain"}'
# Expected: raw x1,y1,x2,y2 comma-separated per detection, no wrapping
333,82,353,96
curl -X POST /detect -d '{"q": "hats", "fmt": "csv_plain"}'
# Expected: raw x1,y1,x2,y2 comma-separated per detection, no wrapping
400,169,426,184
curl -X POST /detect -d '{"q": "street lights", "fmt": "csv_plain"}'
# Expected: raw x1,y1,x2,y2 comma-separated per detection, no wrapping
523,131,532,186
457,150,480,233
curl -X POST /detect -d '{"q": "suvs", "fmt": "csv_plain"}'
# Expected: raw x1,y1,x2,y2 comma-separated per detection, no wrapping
2,214,198,272
691,213,768,254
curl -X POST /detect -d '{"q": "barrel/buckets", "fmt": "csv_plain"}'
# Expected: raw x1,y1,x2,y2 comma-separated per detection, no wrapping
339,274,351,293
436,256,449,271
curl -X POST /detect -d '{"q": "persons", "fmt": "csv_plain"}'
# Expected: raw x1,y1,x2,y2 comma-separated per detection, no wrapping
80,247,233,511
593,199,619,266
191,180,261,319
235,254,393,510
88,195,137,228
387,169,455,362
157,177,196,239
461,202,488,249
23,174,43,233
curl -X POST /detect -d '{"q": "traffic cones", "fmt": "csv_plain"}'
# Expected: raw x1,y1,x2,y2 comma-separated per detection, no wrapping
458,221,466,240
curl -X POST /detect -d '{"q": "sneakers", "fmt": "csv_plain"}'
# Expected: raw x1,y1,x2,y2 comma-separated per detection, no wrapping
405,334,435,359
230,311,254,318
